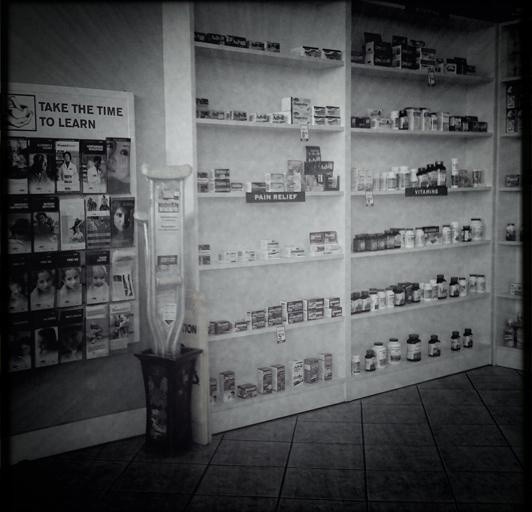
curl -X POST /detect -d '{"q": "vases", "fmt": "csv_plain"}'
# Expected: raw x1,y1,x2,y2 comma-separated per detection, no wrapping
133,346,203,458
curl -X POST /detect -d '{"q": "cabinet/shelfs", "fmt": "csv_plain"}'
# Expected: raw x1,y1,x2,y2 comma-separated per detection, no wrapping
161,1,523,444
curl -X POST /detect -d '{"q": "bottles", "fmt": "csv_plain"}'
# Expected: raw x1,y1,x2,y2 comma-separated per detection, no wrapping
450,331,461,351
462,328,473,349
503,313,524,348
387,338,402,365
428,334,441,358
506,223,515,241
398,217,484,249
351,158,482,192
364,341,387,372
351,274,488,314
391,109,408,131
351,355,363,375
406,333,422,362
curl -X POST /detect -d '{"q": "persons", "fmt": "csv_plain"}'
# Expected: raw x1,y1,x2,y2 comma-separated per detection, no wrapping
3,138,133,369
